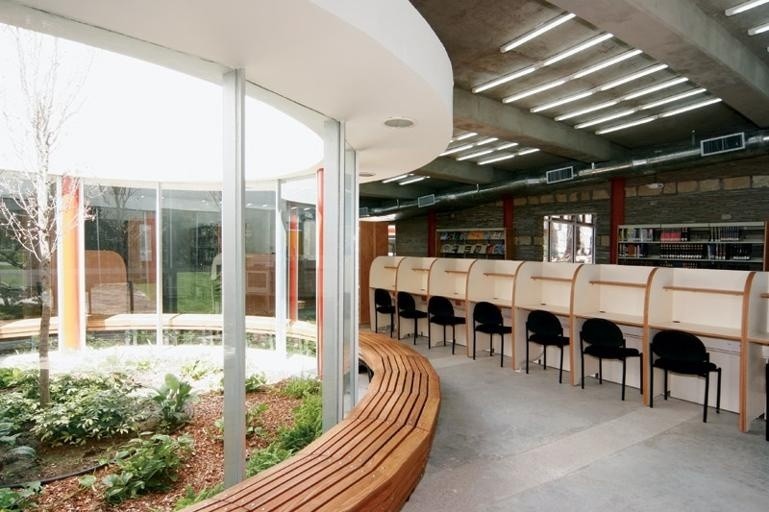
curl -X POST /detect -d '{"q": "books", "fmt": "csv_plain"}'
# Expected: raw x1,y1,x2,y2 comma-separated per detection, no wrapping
618,227,751,268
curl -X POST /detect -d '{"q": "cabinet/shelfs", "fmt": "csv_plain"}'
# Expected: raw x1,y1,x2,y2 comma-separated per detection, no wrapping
616,220,769,272
434,227,507,260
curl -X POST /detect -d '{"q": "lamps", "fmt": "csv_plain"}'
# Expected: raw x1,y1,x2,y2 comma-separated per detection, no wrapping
723,0,769,36
381,175,428,186
